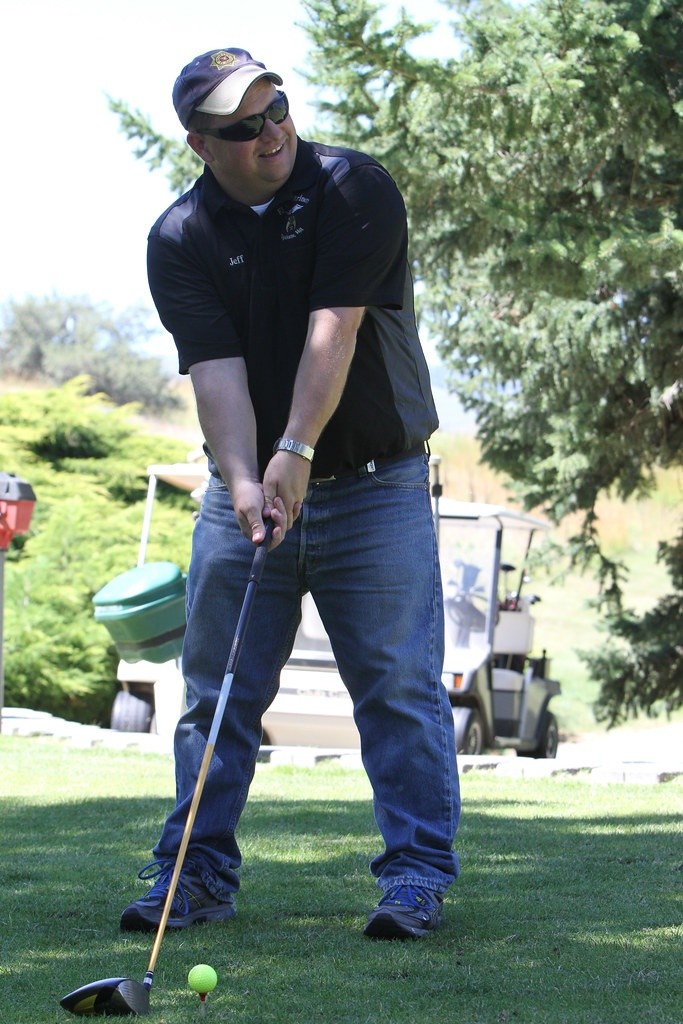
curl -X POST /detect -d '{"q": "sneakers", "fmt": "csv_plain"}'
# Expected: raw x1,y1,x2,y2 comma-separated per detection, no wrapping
119,858,236,929
363,884,443,940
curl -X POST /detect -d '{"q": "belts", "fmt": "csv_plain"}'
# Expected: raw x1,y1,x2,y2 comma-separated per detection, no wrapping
309,442,426,484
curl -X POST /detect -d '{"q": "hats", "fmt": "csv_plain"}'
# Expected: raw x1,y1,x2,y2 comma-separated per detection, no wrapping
173,48,283,129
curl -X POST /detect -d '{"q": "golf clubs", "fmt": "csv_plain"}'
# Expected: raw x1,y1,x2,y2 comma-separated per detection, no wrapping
58,517,277,1017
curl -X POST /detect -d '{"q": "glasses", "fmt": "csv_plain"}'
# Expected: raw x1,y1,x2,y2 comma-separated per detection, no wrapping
191,90,289,142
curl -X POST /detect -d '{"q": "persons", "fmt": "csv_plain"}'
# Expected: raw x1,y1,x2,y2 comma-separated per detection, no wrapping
119,47,462,938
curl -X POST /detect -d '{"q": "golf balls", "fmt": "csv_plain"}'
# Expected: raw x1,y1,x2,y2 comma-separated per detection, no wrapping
187,963,218,994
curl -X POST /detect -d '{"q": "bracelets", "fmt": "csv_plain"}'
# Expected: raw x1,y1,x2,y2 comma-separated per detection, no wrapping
272,438,315,462
